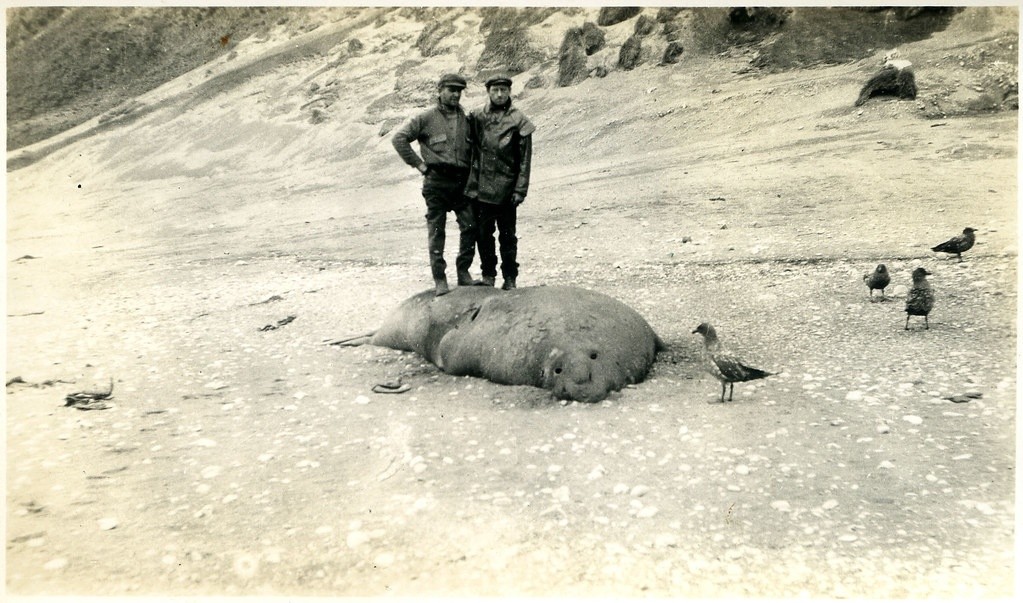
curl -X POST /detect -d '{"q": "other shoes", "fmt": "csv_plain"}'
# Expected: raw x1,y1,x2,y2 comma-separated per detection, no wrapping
434,274,449,297
457,268,485,286
483,275,495,287
503,276,516,290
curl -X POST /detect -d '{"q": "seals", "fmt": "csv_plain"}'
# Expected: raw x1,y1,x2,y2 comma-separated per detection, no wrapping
331,282,671,404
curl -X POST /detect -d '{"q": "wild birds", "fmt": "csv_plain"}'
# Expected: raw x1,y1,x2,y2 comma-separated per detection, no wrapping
691,322,782,404
904,268,936,331
930,227,979,264
862,264,892,303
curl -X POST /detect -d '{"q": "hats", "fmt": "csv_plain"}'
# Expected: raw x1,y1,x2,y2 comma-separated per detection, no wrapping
485,74,512,87
438,73,466,88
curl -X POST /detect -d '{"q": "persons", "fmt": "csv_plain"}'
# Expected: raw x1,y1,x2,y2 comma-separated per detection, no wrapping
392,73,536,297
